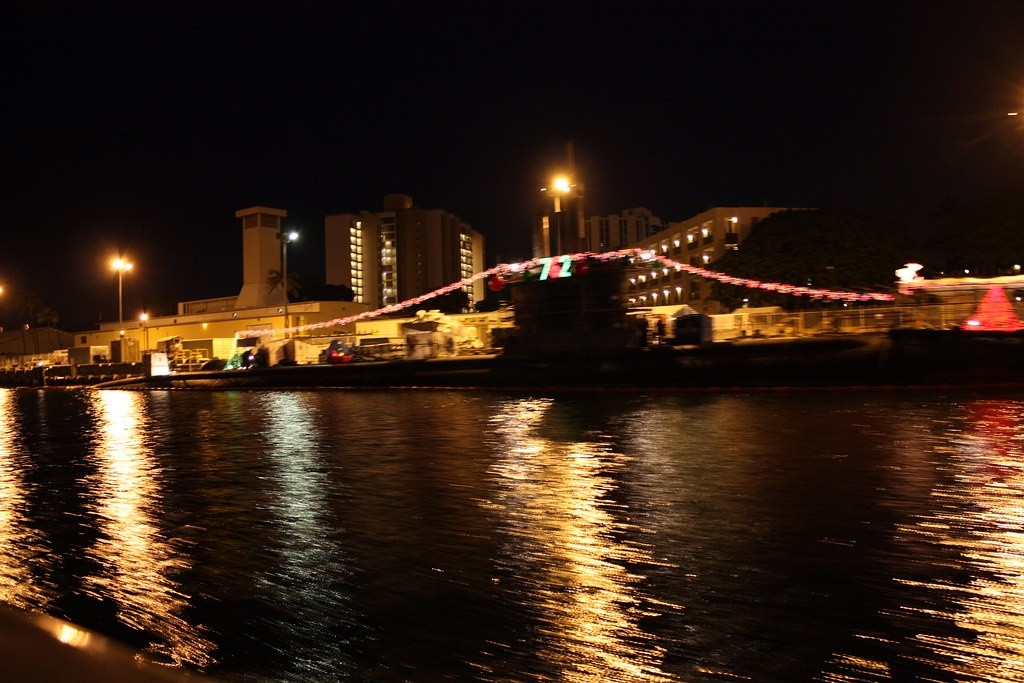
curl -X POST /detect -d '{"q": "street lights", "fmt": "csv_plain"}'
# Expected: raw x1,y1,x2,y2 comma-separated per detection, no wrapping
112,259,132,338
550,176,571,259
284,231,299,338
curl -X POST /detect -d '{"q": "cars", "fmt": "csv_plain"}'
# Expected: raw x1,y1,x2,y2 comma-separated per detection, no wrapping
322,340,353,363
